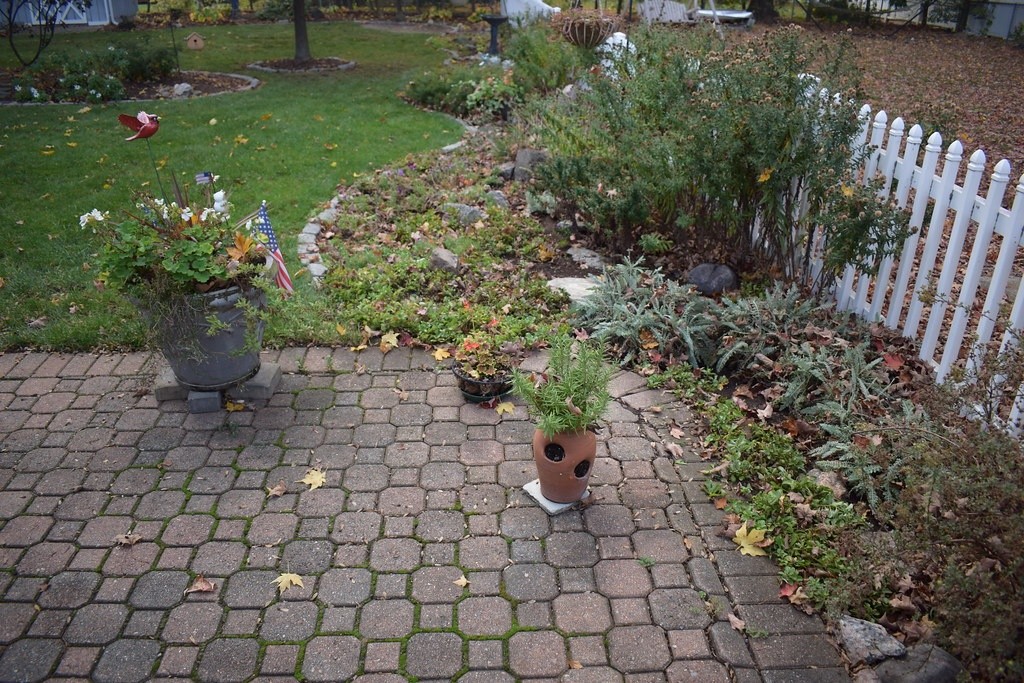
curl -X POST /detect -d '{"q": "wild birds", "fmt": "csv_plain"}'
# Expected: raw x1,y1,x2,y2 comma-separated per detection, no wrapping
118,111,161,141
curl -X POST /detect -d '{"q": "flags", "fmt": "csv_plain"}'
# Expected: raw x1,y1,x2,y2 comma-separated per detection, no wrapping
195,171,213,184
255,200,294,297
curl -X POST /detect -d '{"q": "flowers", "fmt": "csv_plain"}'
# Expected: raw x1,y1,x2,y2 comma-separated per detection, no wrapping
78,112,277,287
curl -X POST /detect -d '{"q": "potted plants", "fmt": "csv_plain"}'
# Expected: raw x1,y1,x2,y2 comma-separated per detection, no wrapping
452,329,518,404
515,326,616,504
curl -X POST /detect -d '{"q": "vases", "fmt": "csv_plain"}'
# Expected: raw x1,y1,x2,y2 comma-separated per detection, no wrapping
119,291,266,393
